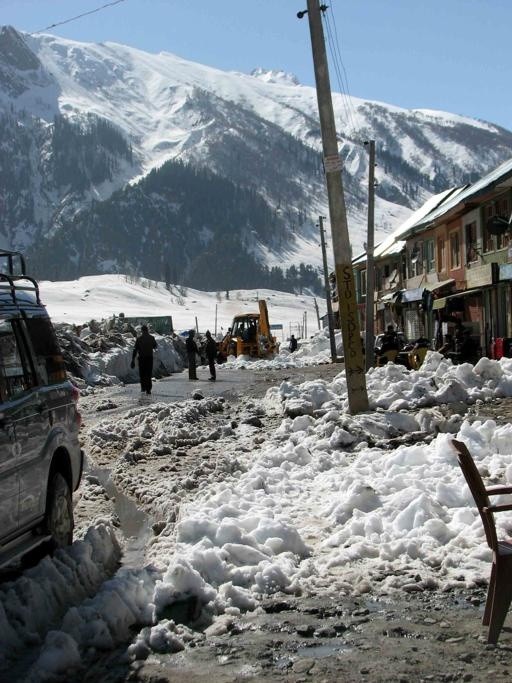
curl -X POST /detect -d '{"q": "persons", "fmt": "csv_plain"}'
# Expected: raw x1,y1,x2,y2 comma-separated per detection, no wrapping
185,331,201,381
374,325,398,355
289,335,298,353
199,329,217,382
130,326,158,395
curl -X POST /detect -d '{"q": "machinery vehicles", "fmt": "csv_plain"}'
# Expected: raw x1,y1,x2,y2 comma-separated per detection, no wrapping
376,326,432,370
218,300,280,359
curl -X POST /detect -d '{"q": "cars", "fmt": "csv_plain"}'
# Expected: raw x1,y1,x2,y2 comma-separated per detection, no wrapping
0,248,84,586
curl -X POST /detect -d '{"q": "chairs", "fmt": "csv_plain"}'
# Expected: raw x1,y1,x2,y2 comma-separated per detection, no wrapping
446,438,512,646
